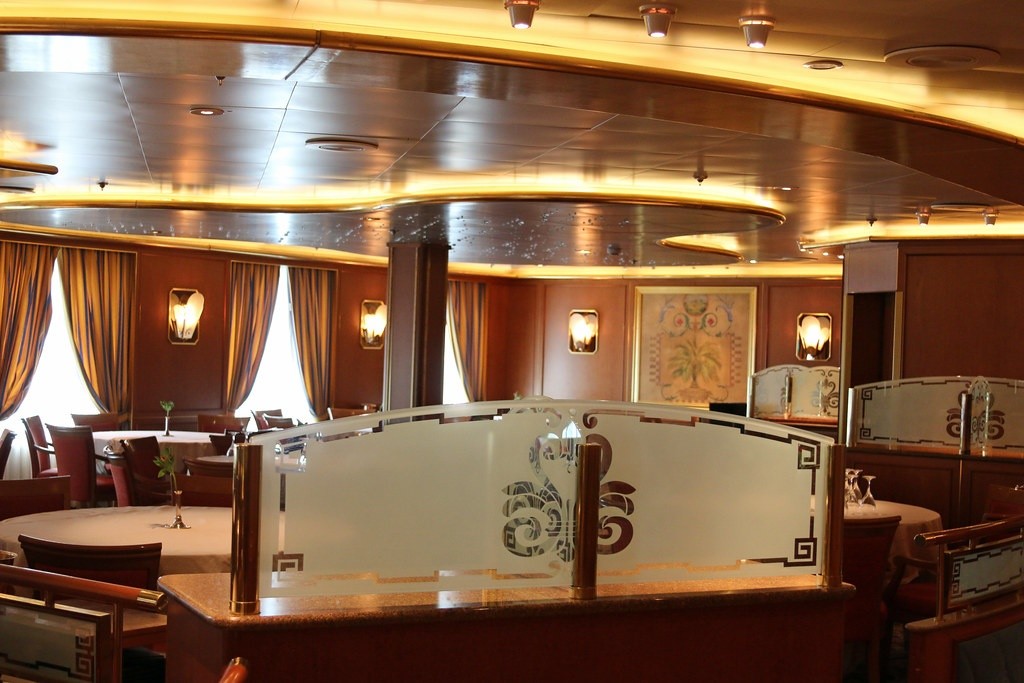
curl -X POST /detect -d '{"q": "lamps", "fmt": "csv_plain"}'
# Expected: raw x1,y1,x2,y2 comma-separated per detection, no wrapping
915,211,930,225
738,16,776,49
168,288,205,344
504,0,540,30
795,311,833,361
638,4,676,38
982,213,999,225
360,300,388,349
568,309,600,356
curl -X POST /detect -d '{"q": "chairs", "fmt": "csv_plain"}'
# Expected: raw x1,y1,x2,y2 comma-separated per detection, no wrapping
841,516,939,683
0,409,377,613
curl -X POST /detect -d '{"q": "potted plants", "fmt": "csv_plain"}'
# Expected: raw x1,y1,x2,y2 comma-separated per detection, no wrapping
154,447,187,529
160,400,175,436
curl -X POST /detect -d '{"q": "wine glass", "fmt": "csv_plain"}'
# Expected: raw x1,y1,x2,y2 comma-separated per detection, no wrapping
844,468,876,512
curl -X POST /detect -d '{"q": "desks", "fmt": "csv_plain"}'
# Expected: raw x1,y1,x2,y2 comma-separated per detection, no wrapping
92,429,225,475
0,505,286,598
844,499,945,591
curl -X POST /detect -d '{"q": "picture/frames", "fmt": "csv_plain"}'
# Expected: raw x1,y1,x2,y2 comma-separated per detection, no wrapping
631,286,757,411
